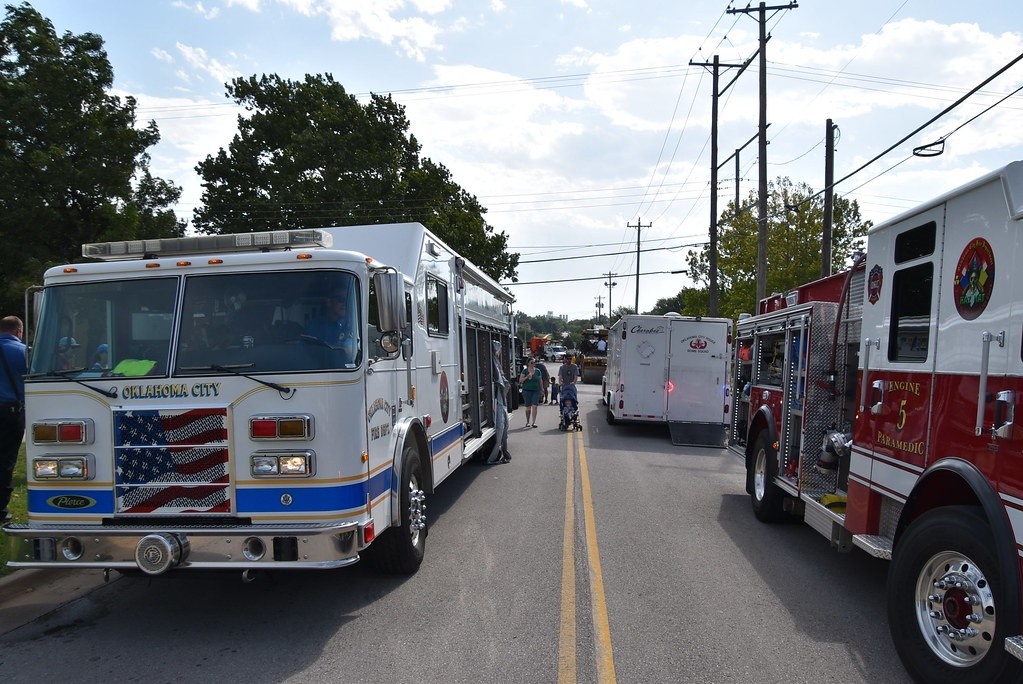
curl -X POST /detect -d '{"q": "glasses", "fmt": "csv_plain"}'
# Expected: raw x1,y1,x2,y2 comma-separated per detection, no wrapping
566,358,573,361
530,362,536,365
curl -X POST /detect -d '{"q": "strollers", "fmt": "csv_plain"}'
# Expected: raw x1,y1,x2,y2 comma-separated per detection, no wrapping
557,381,583,431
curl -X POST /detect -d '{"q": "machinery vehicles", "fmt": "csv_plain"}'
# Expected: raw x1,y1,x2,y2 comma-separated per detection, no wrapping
571,324,608,383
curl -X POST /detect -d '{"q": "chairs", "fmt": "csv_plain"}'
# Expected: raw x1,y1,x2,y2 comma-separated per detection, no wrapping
561,396,579,422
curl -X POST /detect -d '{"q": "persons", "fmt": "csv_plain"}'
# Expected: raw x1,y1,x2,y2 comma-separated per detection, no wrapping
0,315,109,520
519,354,580,431
304,281,360,356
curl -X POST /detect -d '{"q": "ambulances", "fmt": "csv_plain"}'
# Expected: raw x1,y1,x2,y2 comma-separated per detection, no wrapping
601,313,734,448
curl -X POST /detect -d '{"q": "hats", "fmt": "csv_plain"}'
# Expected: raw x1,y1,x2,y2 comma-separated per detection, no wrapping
96,344,108,353
59,337,80,346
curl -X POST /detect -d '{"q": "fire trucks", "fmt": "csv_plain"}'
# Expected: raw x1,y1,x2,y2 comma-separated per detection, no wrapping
727,157,1023,684
6,221,517,587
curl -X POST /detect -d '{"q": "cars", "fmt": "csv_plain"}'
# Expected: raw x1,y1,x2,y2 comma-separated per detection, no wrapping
544,345,567,363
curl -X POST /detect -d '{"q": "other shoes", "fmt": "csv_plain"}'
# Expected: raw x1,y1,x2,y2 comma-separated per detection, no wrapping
544,400,548,404
539,400,543,404
526,423,530,427
555,401,558,405
4,514,12,521
532,424,538,428
549,402,553,405
